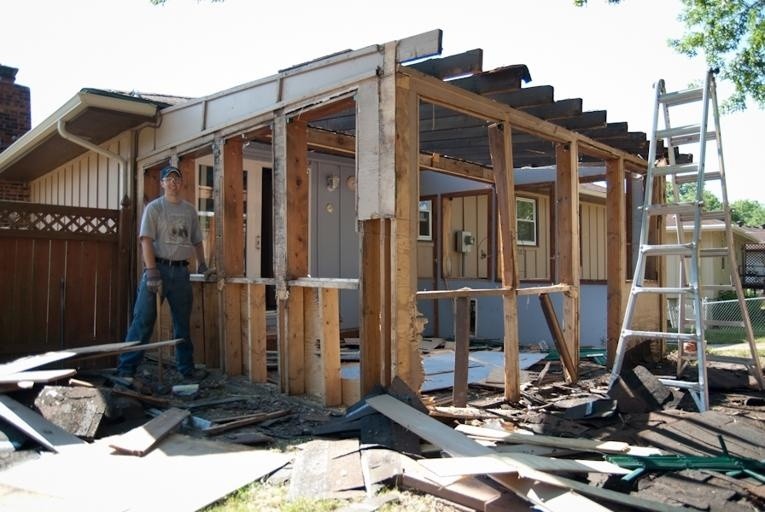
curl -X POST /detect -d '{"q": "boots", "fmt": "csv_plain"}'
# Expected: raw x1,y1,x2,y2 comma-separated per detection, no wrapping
177,361,208,381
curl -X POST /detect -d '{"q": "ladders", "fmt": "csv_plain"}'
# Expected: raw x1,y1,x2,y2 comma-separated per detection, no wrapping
608,68,765,412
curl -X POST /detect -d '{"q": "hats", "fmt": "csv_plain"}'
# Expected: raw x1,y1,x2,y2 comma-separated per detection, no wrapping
159,166,182,179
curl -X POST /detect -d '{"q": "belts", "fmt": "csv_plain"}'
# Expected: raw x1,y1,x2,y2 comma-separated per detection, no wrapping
155,257,189,267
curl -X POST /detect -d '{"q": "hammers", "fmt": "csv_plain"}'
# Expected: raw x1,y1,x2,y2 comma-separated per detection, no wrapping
152,292,171,394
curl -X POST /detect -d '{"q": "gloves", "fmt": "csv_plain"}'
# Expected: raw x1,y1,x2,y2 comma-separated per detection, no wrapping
145,269,162,293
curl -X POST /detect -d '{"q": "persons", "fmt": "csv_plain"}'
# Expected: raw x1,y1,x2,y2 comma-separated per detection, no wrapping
116,166,212,382
